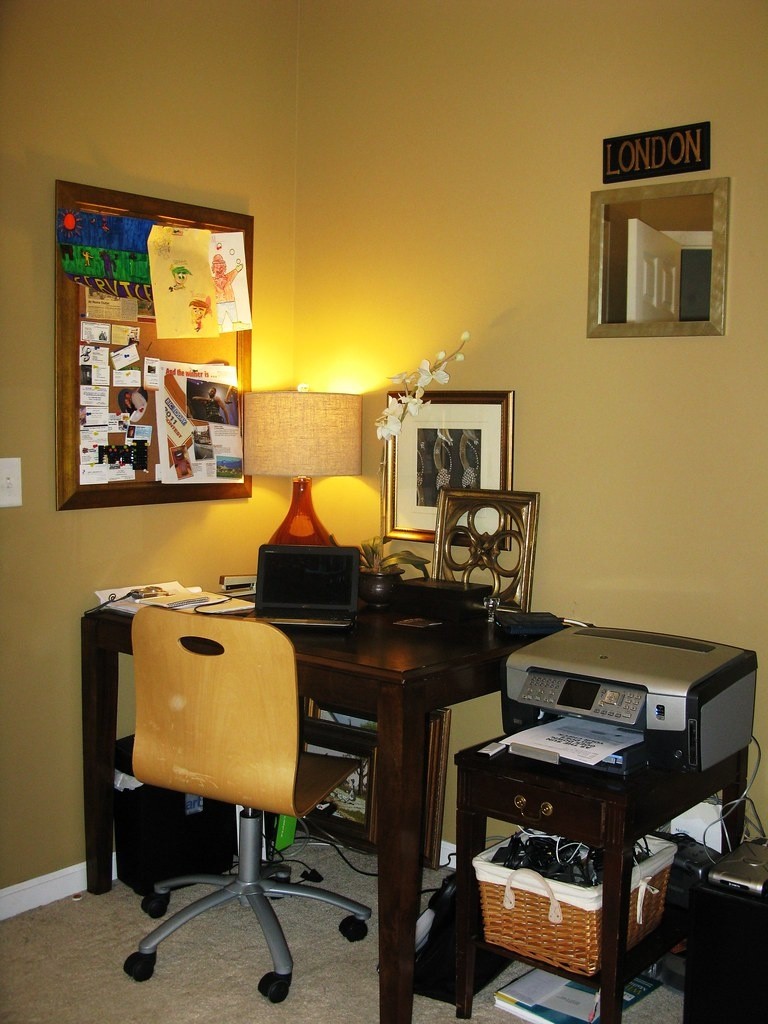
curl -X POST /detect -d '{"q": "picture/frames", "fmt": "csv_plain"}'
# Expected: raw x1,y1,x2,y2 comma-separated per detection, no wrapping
384,390,516,552
431,486,541,614
297,696,452,872
54,178,253,512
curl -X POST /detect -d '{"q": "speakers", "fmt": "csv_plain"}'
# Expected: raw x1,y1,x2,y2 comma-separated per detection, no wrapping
682,878,768,1024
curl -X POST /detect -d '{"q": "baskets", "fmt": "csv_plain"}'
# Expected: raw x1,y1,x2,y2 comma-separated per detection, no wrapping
472,827,678,977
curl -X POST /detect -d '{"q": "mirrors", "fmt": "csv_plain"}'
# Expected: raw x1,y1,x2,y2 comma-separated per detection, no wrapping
586,176,725,338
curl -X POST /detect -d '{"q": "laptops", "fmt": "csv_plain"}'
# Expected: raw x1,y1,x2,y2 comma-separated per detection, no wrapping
245,544,361,629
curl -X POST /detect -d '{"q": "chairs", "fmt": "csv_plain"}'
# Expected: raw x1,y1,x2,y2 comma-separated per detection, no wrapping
122,606,373,1004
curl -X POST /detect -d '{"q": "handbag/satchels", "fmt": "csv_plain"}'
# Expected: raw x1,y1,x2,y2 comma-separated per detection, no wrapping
376,873,515,1006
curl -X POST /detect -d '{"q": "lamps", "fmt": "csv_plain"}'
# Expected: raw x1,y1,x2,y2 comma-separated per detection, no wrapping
243,382,362,545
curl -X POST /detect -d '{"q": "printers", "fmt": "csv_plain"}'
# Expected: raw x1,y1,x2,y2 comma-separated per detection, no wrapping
499,625,759,783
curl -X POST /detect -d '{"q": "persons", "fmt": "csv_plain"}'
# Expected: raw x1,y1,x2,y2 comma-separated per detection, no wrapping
192,387,229,424
128,426,134,438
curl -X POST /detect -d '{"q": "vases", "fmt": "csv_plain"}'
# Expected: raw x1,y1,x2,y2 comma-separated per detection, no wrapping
358,569,406,609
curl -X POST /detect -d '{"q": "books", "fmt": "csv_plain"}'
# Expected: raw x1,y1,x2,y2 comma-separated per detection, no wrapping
494,969,663,1024
95,581,255,614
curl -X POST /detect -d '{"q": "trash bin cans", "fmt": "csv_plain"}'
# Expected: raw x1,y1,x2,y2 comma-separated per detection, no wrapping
116,733,239,897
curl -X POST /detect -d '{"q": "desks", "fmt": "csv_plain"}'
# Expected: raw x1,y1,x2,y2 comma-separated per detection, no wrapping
683,881,768,1024
452,736,749,1024
80,589,572,1024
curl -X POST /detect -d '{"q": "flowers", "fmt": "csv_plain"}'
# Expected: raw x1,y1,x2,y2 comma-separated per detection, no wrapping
328,330,470,579
